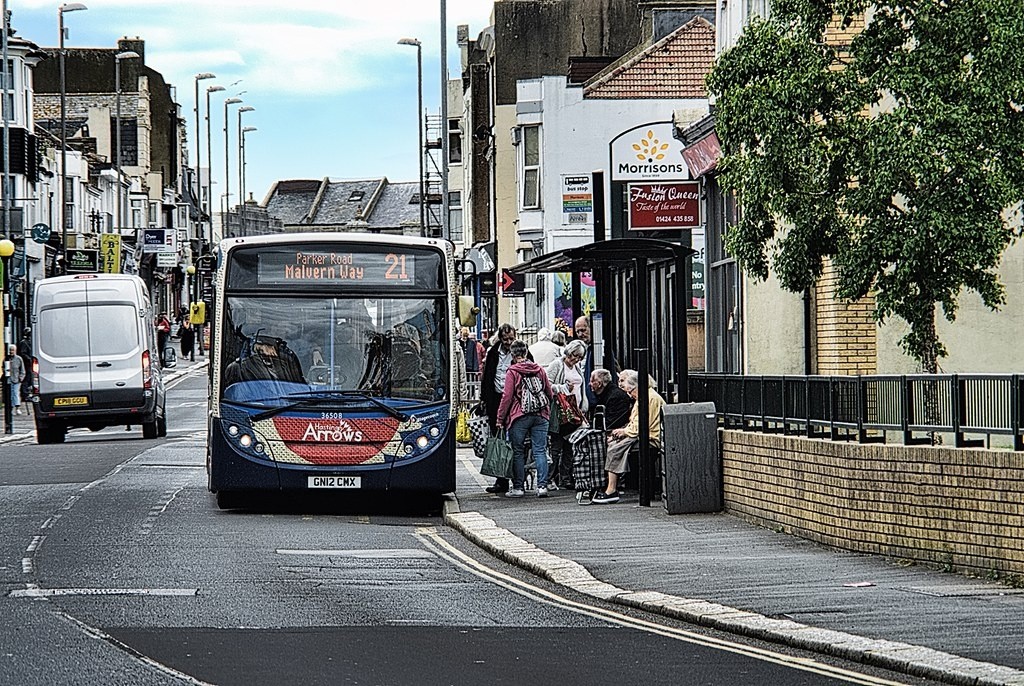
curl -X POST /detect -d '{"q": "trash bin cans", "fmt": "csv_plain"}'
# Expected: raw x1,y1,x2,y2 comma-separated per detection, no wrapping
663,401,724,516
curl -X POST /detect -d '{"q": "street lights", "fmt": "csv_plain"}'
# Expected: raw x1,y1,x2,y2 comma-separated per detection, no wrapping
56,2,88,275
237,105,256,237
222,97,244,239
220,192,235,240
395,36,427,239
115,51,140,235
241,125,259,236
204,85,228,257
193,72,217,268
0,239,17,435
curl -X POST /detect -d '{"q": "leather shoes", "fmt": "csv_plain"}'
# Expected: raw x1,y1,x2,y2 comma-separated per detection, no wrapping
486,485,509,493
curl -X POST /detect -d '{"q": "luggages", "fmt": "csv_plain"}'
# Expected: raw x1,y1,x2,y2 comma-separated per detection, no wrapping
568,404,608,505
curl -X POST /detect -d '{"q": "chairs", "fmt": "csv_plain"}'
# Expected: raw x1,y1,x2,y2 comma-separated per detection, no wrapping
230,308,438,394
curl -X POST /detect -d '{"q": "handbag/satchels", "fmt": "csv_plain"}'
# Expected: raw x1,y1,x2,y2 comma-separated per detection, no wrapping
480,426,514,480
457,402,473,444
549,388,582,435
467,414,494,458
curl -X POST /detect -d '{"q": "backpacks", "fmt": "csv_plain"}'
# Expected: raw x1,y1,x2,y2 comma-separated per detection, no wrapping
513,368,550,413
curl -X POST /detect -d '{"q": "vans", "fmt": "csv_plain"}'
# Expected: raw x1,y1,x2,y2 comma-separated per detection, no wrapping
31,271,176,446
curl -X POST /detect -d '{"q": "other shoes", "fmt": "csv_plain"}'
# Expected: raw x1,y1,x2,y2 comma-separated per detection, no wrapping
16,408,22,414
559,476,576,490
547,482,556,491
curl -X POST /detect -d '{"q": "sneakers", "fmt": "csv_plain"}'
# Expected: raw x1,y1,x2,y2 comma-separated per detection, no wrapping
592,491,620,503
504,489,524,497
537,488,548,497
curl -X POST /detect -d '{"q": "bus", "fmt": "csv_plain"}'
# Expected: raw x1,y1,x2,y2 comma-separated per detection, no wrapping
187,232,481,511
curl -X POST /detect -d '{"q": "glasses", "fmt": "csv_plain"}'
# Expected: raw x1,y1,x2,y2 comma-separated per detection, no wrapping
627,387,635,394
573,352,582,361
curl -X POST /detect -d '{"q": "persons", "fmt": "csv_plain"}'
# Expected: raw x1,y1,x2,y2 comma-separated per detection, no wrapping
456,313,668,502
6,327,31,416
272,319,323,365
154,302,193,360
